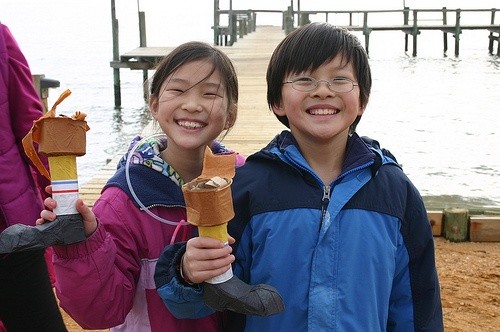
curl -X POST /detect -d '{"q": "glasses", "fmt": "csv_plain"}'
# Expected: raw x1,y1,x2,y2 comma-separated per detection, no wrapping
281,76,359,93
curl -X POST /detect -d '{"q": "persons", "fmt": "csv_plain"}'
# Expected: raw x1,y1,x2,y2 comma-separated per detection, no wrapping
36,40,247,332
0,23,68,332
155,23,444,332
